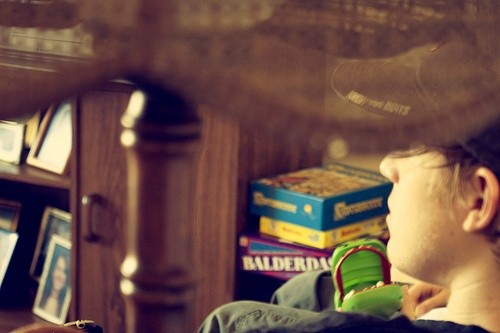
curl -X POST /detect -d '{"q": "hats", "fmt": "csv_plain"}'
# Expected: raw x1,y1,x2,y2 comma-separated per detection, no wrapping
331,32,500,175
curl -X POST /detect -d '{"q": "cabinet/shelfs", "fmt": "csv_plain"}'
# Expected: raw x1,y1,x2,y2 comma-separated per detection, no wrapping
0,43,323,333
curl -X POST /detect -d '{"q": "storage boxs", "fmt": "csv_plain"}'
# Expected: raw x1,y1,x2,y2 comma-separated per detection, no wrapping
247,164,393,231
259,216,388,250
238,230,390,283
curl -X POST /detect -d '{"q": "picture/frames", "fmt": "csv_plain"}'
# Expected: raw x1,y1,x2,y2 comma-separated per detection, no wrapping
28,206,72,281
31,234,72,325
0,199,22,233
26,100,74,175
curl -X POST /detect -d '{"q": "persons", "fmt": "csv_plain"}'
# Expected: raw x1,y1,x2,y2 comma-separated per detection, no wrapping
198,125,500,333
38,253,70,319
